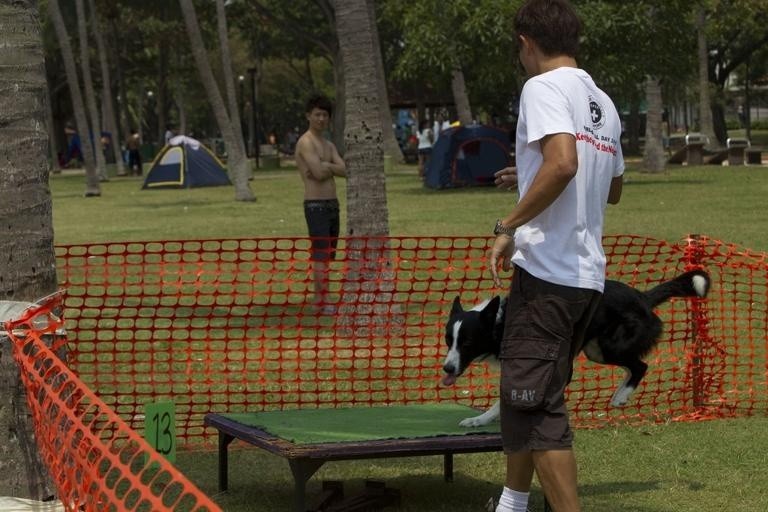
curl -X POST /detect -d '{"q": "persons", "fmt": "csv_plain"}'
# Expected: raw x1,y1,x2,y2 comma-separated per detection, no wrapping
435,107,451,131
487,0,625,512
122,130,145,176
413,116,437,180
296,88,348,311
164,123,174,146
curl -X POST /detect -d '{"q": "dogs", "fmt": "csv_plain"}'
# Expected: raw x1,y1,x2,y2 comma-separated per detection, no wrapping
442,268,712,429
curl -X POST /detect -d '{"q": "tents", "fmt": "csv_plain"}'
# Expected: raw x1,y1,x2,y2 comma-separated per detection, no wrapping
422,122,513,192
136,135,233,190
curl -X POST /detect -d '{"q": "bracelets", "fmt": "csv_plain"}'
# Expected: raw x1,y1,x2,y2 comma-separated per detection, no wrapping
492,219,517,239
